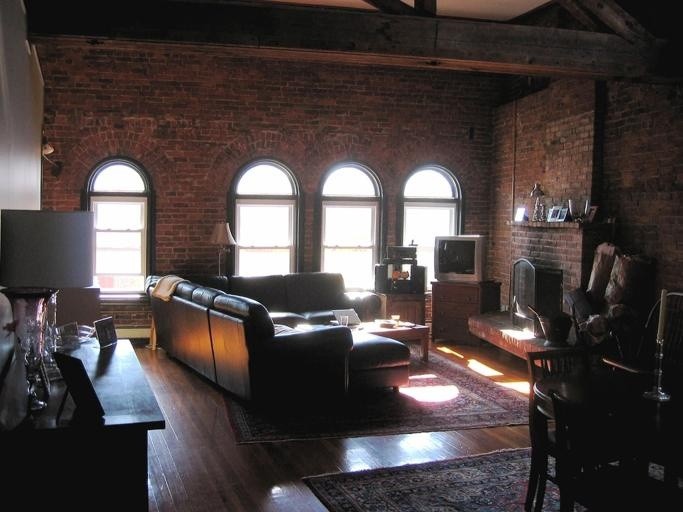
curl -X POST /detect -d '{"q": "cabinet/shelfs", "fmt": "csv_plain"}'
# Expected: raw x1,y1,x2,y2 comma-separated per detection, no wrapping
375,292,425,325
431,280,495,343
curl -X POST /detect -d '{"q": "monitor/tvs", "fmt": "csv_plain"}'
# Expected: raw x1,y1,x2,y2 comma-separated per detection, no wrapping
434,235,489,284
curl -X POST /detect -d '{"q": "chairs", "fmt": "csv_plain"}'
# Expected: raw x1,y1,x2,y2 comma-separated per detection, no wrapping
524,291,683,512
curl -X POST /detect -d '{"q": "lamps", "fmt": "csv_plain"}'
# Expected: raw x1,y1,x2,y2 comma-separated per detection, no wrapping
208,222,238,278
42,142,63,176
0,210,89,383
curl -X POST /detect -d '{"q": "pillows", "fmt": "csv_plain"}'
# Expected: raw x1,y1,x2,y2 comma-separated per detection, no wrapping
148,272,341,336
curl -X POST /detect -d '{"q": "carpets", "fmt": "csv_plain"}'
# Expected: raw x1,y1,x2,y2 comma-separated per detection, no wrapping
222,348,529,447
301,449,681,512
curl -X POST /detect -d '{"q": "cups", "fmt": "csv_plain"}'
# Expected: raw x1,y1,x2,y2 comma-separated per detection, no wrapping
339,315,348,327
390,314,400,327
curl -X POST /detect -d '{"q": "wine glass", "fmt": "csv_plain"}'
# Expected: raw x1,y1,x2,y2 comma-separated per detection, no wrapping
0,283,59,432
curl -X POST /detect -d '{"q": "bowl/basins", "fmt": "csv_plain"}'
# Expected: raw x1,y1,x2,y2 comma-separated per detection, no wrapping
536,312,573,347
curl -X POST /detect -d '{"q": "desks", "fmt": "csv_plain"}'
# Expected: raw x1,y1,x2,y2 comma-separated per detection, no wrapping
38,339,166,512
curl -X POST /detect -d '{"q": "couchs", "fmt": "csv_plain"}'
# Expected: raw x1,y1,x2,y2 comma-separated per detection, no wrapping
147,286,411,413
